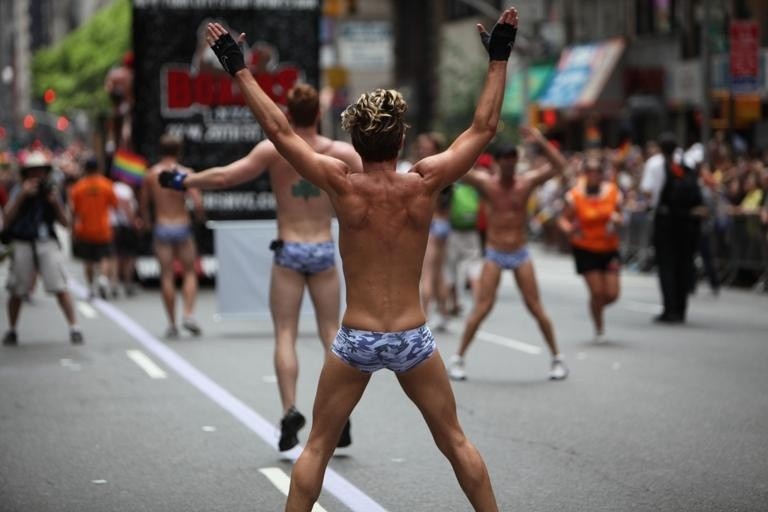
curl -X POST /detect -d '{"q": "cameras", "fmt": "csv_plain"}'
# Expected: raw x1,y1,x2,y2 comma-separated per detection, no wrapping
40,178,55,192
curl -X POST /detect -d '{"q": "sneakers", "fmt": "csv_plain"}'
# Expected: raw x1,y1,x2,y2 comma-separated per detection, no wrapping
337,416,352,448
4,329,17,346
418,303,464,334
654,307,686,323
166,316,203,339
447,353,469,379
70,331,82,346
595,328,607,345
549,352,571,379
86,273,138,300
279,409,306,452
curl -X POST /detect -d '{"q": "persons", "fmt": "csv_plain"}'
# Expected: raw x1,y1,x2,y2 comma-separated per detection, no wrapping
204,6,518,511
0,131,211,340
447,125,567,381
159,83,365,451
413,125,767,340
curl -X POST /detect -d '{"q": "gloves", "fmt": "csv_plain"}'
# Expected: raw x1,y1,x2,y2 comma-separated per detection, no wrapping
209,32,246,77
157,169,189,190
479,23,518,62
